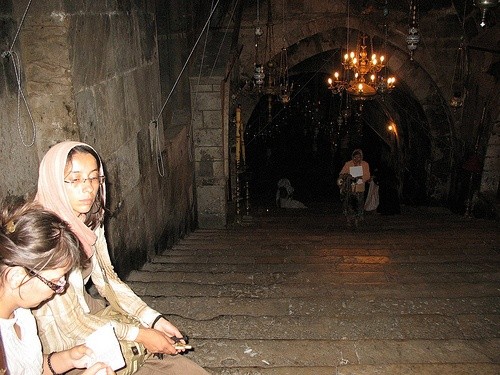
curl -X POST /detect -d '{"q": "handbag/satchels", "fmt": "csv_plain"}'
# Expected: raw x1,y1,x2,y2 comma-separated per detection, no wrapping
364,180,379,211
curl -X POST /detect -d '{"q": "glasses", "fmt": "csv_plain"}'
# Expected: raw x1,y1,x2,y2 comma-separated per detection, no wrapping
6,263,67,294
64,175,106,189
353,157,361,161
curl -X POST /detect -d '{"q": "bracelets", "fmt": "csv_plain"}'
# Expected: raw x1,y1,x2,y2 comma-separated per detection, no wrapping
152,316,169,330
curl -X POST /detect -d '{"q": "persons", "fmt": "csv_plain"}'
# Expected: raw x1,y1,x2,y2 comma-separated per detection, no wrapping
338,149,370,221
47,352,58,375
276,175,308,208
0,195,116,375
30,140,213,375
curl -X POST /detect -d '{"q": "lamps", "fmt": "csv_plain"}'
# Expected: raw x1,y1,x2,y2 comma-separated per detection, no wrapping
327,33,398,112
